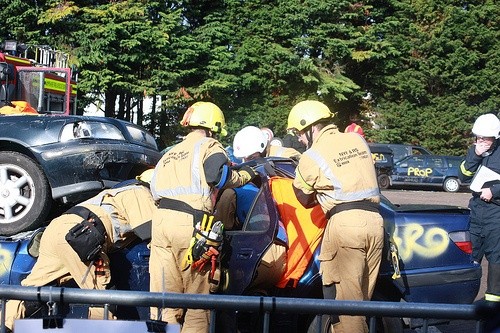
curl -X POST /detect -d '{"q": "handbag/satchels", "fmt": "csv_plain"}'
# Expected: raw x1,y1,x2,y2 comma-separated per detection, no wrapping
64,220,107,263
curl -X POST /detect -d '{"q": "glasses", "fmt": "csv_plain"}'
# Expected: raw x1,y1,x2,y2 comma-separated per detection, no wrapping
292,126,311,138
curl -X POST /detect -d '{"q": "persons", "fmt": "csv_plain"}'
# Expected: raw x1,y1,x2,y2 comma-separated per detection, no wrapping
0,100,385,333
457,113,500,304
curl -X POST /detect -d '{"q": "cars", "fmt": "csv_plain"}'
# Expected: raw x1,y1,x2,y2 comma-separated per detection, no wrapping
0,148,483,333
1,113,164,238
370,144,475,194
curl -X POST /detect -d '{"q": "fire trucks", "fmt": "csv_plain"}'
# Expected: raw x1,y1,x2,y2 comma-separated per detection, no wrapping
0,49,80,115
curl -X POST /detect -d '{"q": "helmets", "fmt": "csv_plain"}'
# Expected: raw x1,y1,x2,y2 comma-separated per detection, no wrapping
180,102,225,134
286,99,334,133
471,113,500,139
136,168,155,186
345,123,365,138
233,125,268,159
